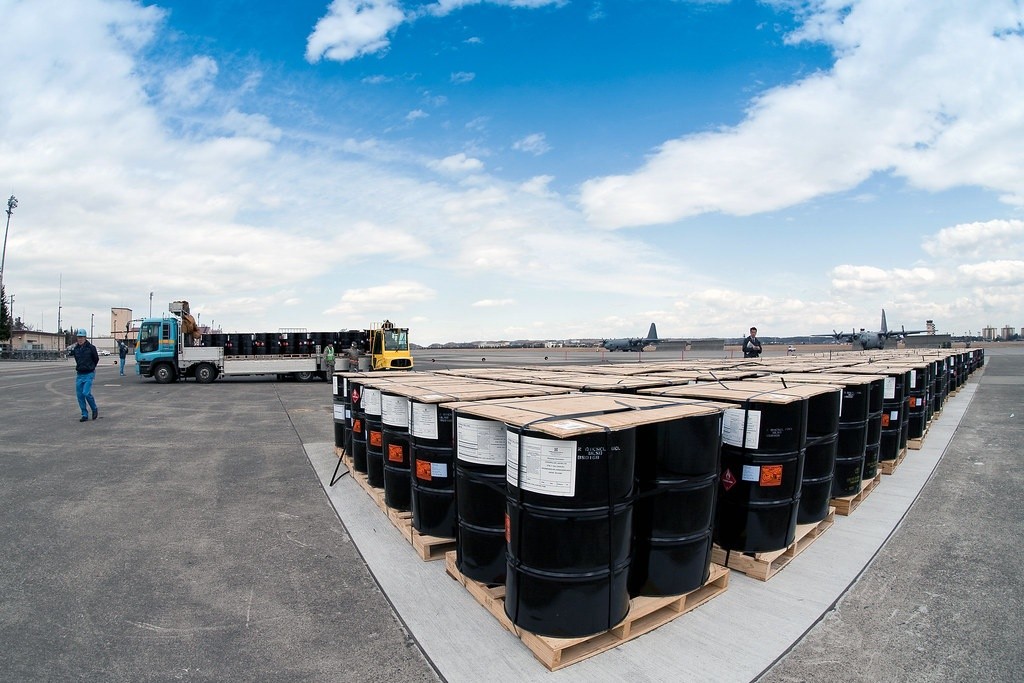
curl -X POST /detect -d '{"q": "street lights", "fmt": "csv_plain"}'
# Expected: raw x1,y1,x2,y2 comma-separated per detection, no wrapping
0,196,18,287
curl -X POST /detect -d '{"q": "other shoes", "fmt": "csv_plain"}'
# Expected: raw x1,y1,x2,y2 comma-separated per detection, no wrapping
79,415,88,422
120,374,127,377
92,408,98,420
327,380,333,384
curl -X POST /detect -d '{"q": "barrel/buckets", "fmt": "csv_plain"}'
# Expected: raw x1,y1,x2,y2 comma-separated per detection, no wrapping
200,331,381,355
332,346,990,638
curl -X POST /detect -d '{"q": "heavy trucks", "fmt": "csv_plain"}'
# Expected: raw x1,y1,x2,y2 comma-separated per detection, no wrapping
133,300,398,384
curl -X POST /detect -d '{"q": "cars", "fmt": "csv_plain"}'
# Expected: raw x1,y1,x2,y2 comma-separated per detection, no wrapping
96,348,111,356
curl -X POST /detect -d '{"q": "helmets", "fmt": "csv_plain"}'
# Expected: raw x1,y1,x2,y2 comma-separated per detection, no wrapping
77,329,86,336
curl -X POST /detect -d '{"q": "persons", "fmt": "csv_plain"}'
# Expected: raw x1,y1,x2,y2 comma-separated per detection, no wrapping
742,327,762,358
348,341,359,372
324,341,335,384
119,342,128,376
74,329,99,422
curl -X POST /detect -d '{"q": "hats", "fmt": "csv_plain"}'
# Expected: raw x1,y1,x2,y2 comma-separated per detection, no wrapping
351,341,357,345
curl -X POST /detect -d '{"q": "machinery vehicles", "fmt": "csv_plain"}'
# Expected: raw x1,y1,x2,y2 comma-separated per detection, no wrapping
371,320,413,371
